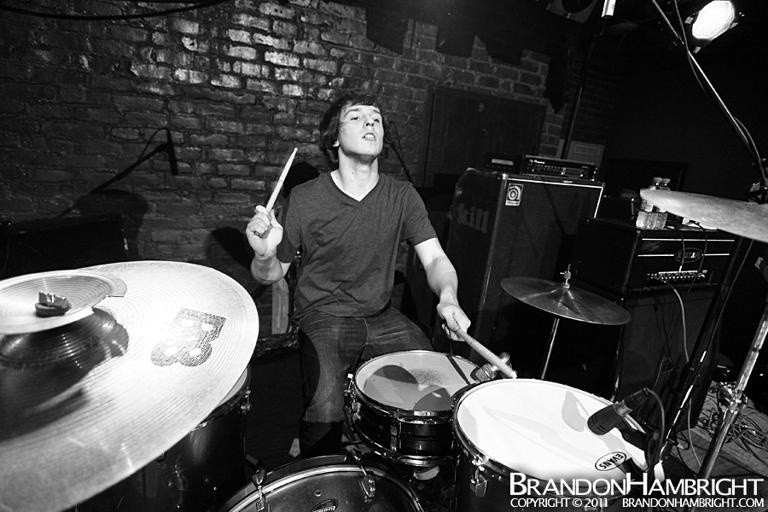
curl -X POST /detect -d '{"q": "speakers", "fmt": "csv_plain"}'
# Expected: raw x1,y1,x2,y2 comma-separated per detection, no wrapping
539,282,723,436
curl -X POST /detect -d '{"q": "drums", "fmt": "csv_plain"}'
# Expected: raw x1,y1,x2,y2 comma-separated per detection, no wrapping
76,367,252,511
341,350,484,470
180,422,425,512
436,377,645,512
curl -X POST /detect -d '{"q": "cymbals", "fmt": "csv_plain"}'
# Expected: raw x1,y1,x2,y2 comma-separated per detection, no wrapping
641,190,768,246
0,259,260,512
499,275,633,327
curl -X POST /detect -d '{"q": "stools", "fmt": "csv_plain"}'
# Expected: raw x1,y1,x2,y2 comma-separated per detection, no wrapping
297,336,318,409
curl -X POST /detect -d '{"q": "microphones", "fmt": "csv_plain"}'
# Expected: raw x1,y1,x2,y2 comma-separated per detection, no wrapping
601,0,616,19
588,388,650,435
166,131,179,176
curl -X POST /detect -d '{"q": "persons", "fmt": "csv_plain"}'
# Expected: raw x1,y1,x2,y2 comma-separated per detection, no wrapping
243,94,471,494
